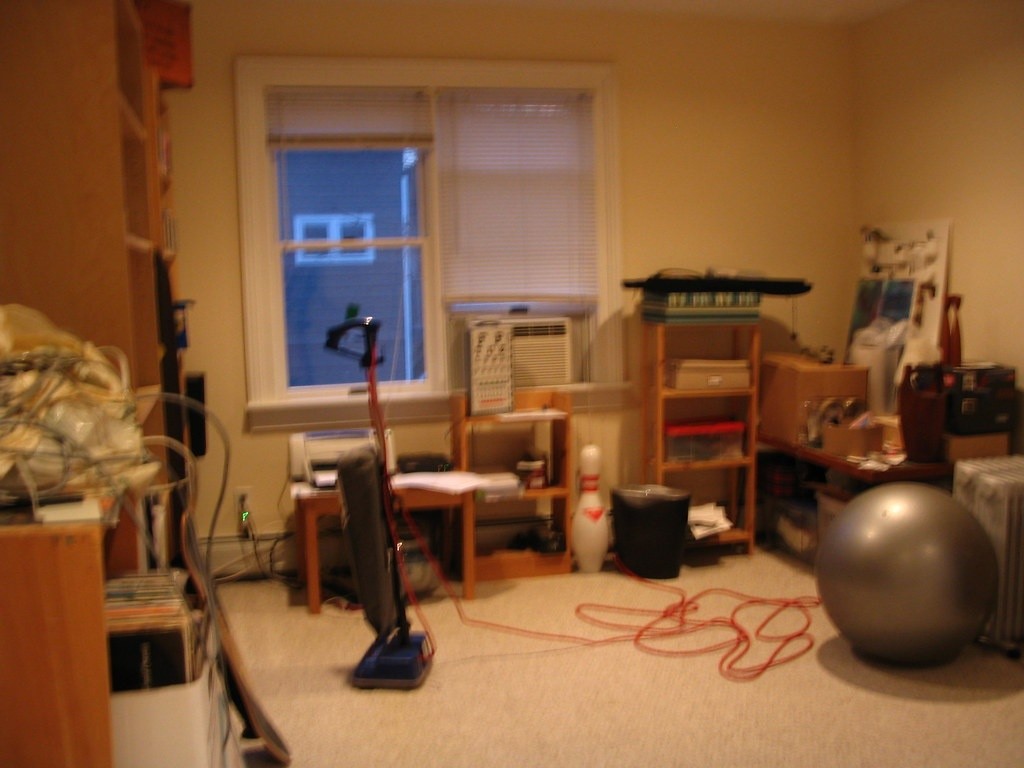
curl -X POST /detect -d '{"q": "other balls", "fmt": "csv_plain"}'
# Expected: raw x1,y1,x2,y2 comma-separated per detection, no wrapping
811,481,1000,667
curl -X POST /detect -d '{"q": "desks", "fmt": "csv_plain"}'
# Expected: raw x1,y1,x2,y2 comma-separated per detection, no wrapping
760,436,953,484
296,474,474,614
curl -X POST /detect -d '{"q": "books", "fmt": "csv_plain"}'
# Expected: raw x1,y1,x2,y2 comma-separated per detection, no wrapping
104,571,193,694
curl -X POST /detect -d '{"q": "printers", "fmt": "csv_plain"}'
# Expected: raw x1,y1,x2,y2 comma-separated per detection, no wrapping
288,426,397,488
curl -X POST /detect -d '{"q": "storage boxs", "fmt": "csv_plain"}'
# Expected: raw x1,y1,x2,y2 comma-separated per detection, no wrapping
104,616,224,768
761,352,869,447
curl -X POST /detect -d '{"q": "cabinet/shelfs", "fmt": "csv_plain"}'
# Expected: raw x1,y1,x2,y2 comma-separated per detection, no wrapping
452,399,573,582
0,477,157,768
0,0,188,547
645,318,757,558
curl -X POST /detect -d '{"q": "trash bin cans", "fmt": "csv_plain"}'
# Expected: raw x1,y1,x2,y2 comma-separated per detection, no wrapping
611,484,691,580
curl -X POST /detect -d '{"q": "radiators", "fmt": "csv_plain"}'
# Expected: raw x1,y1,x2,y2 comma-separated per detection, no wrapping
953,455,1024,664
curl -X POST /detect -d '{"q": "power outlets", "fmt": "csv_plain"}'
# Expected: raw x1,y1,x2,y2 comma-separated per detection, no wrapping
234,485,250,506
